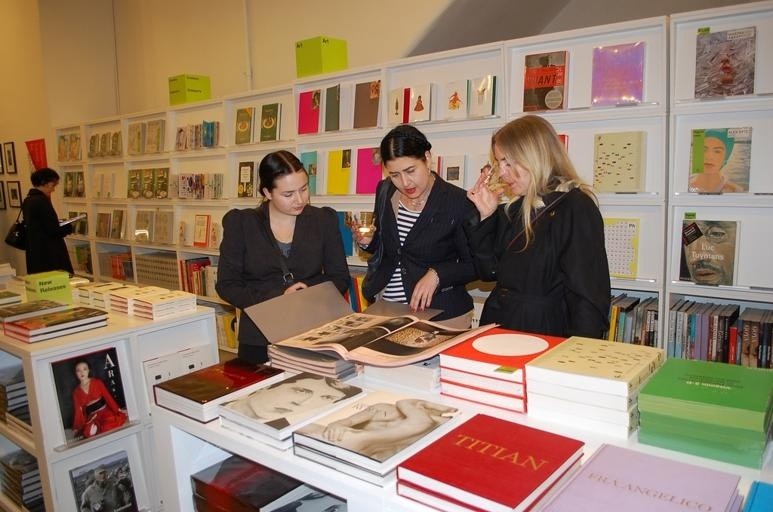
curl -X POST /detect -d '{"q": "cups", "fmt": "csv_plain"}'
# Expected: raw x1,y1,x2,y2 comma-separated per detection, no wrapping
471,162,515,205
353,212,376,246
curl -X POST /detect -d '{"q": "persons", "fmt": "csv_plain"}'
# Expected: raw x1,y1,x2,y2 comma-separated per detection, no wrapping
458,113,609,341
22,167,75,274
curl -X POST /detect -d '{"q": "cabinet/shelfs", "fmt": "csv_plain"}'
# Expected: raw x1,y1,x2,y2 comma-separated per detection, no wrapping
0,269,217,512
149,355,773,512
50,1,773,364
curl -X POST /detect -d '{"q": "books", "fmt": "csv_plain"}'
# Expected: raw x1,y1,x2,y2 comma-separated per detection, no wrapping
0,262,197,512
607,292,773,369
523,42,648,279
57,76,496,348
153,312,773,512
679,27,758,287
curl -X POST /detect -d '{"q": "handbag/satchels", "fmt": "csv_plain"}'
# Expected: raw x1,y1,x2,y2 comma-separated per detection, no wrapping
362,243,383,304
4,222,26,250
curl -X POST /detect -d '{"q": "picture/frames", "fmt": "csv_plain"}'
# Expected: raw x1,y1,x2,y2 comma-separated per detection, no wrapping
5,181,24,208
0,181,7,209
3,140,16,175
